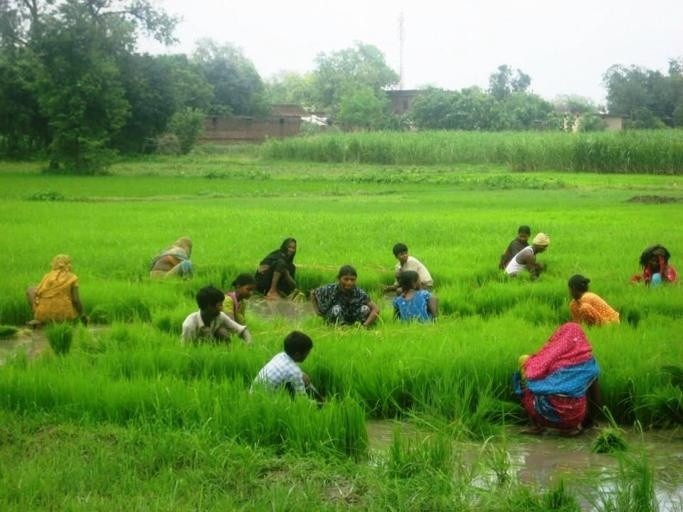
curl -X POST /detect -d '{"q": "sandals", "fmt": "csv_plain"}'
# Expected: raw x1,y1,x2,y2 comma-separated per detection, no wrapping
518,426,546,436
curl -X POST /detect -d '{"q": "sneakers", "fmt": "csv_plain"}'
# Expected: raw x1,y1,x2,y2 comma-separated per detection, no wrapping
568,428,585,437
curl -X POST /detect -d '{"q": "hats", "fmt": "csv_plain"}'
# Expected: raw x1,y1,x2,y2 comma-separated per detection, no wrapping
533,232,550,246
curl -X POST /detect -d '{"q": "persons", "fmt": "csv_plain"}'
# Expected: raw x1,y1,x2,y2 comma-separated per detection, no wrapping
500,226,531,268
182,285,252,344
27,255,87,328
518,322,600,435
384,243,433,296
250,330,312,402
255,237,296,297
505,233,550,277
630,244,678,286
311,264,380,326
151,236,193,281
392,271,436,323
568,274,620,328
221,273,255,326
